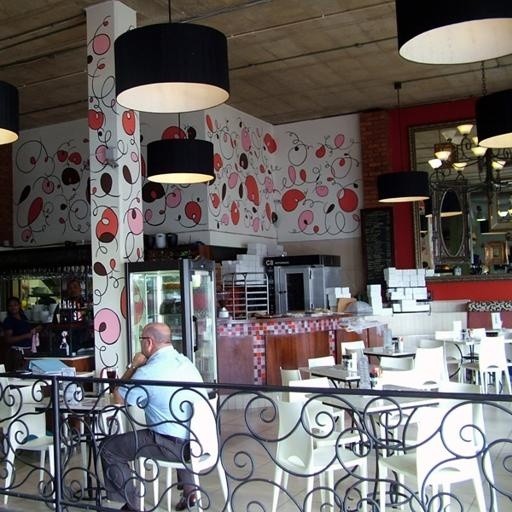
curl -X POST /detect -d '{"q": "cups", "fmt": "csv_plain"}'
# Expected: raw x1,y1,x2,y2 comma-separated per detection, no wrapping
107,368,117,395
23,303,59,322
220,311,230,318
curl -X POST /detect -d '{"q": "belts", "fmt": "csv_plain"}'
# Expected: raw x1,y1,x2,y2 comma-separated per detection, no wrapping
159,434,189,446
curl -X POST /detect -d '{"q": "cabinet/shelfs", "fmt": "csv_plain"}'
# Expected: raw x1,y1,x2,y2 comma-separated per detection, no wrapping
16,244,96,323
221,266,271,319
123,254,219,415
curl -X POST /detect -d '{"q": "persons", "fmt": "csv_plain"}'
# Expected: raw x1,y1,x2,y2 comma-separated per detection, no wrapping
216,298,230,319
1,295,42,372
97,322,220,512
51,278,92,356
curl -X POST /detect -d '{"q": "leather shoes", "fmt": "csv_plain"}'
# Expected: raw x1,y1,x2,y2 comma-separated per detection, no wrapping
175,494,196,511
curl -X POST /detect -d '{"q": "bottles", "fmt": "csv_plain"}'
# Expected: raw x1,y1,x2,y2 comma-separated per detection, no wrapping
63,300,80,320
382,330,404,353
341,354,352,372
460,327,471,341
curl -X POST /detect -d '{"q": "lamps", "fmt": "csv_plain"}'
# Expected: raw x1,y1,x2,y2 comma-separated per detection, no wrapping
389,1,512,73
0,75,23,147
109,0,232,118
372,79,428,206
426,123,510,193
145,111,217,188
470,88,512,150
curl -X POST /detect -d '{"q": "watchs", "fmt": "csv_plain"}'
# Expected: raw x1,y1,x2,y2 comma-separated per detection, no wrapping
125,363,140,370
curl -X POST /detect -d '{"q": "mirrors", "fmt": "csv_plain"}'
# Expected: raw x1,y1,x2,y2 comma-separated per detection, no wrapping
402,108,511,285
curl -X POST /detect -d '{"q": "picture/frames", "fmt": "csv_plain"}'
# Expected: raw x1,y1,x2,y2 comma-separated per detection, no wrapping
482,239,512,268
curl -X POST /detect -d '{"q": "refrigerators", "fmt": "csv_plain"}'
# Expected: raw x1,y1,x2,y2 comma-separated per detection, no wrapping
127,261,221,397
